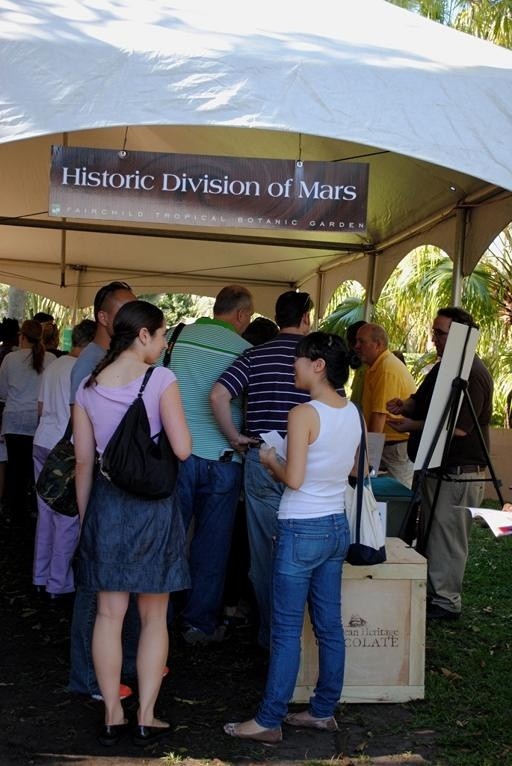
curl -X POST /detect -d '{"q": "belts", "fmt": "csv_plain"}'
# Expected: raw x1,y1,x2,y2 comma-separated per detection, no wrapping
426,465,487,475
384,439,407,446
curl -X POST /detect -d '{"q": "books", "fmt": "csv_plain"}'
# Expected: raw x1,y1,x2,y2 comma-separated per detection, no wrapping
463,507,512,538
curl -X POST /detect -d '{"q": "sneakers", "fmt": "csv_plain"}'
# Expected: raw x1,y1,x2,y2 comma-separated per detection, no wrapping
91,684,133,701
426,603,461,621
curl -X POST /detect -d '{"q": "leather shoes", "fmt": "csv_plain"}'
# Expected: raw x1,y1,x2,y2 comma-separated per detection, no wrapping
99,718,178,746
283,713,339,732
222,722,283,744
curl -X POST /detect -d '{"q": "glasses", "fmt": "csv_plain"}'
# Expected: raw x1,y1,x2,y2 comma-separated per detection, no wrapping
433,328,448,338
97,281,133,311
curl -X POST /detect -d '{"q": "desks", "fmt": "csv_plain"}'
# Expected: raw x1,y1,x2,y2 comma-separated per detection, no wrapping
364,474,414,537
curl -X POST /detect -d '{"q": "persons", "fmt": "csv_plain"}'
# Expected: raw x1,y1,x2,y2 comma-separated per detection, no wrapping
0,281,416,745
385,307,493,621
502,503,512,512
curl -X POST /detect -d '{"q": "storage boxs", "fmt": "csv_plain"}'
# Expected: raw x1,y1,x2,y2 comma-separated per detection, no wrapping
289,536,428,704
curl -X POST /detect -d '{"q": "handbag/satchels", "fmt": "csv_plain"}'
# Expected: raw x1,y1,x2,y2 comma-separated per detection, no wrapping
102,398,179,500
35,437,78,517
344,475,386,566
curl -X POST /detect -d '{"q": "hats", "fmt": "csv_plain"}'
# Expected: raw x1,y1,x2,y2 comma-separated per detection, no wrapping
34,312,54,323
